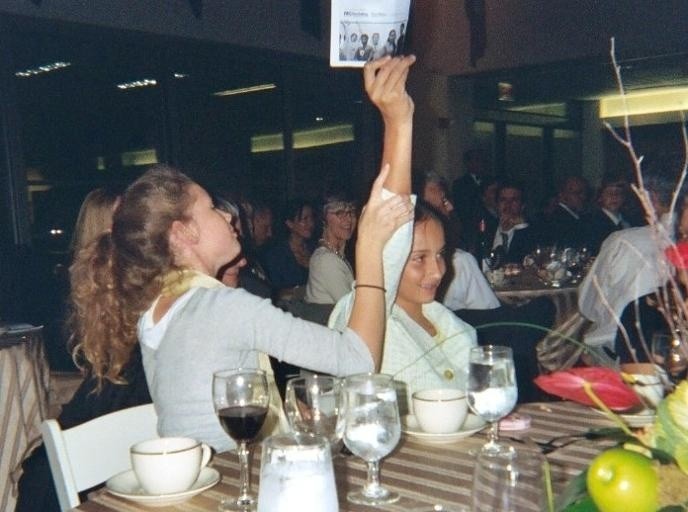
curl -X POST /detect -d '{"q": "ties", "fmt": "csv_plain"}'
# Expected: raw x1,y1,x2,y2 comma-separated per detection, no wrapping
500,232,510,254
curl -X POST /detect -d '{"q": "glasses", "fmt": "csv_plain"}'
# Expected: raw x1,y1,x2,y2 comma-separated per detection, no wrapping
326,209,358,218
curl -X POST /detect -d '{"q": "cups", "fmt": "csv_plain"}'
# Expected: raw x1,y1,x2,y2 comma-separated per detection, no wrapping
256,430,343,512
411,388,475,434
469,437,557,512
283,372,347,460
629,373,663,416
130,435,213,496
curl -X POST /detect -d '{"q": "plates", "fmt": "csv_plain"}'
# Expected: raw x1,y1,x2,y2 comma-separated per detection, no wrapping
394,410,486,444
103,463,221,509
588,400,660,428
0,325,44,335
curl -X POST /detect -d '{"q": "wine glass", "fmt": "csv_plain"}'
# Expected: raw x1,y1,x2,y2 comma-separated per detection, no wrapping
465,343,519,460
652,331,687,384
210,366,269,511
473,238,589,292
336,371,404,506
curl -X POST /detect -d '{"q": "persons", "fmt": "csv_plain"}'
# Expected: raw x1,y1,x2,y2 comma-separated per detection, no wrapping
299,54,486,417
14,186,152,512
66,161,415,457
339,24,408,61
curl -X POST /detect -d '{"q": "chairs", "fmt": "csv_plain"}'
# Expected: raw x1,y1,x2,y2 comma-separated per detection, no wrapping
239,229,688,414
35,397,163,511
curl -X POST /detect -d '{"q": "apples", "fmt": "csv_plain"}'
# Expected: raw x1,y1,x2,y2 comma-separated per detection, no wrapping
587,448,659,511
